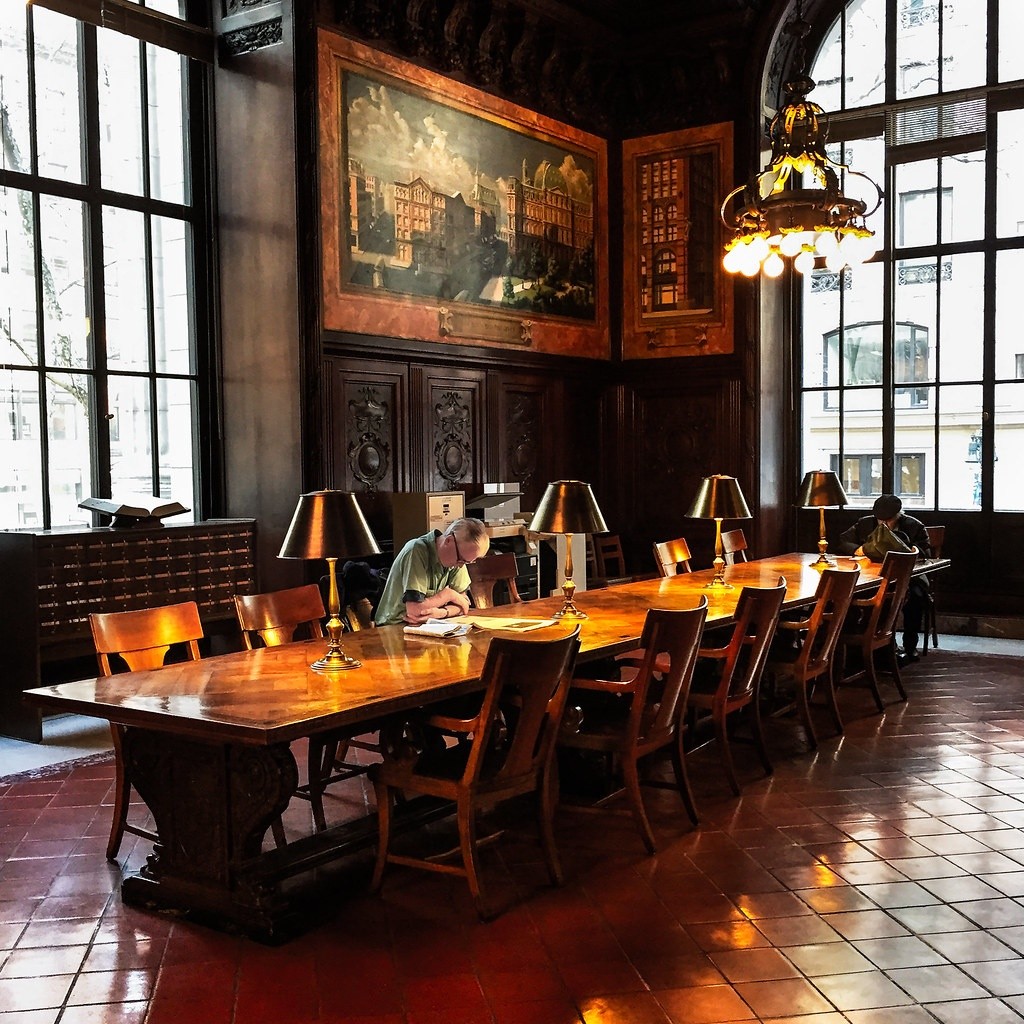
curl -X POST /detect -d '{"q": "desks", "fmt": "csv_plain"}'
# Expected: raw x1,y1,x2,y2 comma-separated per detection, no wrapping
19,552,952,936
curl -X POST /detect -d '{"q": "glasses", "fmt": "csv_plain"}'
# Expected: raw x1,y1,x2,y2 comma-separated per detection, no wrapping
450,531,477,565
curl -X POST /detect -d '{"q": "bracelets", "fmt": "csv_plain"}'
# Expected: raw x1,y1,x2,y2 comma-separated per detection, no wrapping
440,607,449,619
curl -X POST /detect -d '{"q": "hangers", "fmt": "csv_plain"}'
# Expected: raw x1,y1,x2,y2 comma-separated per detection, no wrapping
92,527,946,923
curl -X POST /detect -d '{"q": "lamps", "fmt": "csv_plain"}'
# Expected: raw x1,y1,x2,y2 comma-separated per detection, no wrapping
685,474,756,591
797,471,849,568
530,481,611,620
276,487,383,671
719,0,885,281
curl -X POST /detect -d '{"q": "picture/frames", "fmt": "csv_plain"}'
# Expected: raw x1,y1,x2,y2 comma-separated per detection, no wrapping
310,26,612,364
621,121,736,357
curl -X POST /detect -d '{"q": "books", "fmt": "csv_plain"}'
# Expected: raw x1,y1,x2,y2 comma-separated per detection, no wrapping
78,494,191,520
404,624,460,637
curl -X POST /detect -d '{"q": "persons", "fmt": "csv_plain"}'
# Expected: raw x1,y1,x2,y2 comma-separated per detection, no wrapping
840,495,931,662
375,518,489,756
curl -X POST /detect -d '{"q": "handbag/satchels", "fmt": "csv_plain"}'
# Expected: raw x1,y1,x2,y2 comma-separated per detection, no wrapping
863,523,911,564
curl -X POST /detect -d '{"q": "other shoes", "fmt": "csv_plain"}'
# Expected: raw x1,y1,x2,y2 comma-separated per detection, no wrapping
906,648,919,660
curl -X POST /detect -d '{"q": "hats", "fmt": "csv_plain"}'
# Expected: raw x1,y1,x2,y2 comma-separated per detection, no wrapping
872,494,902,519
